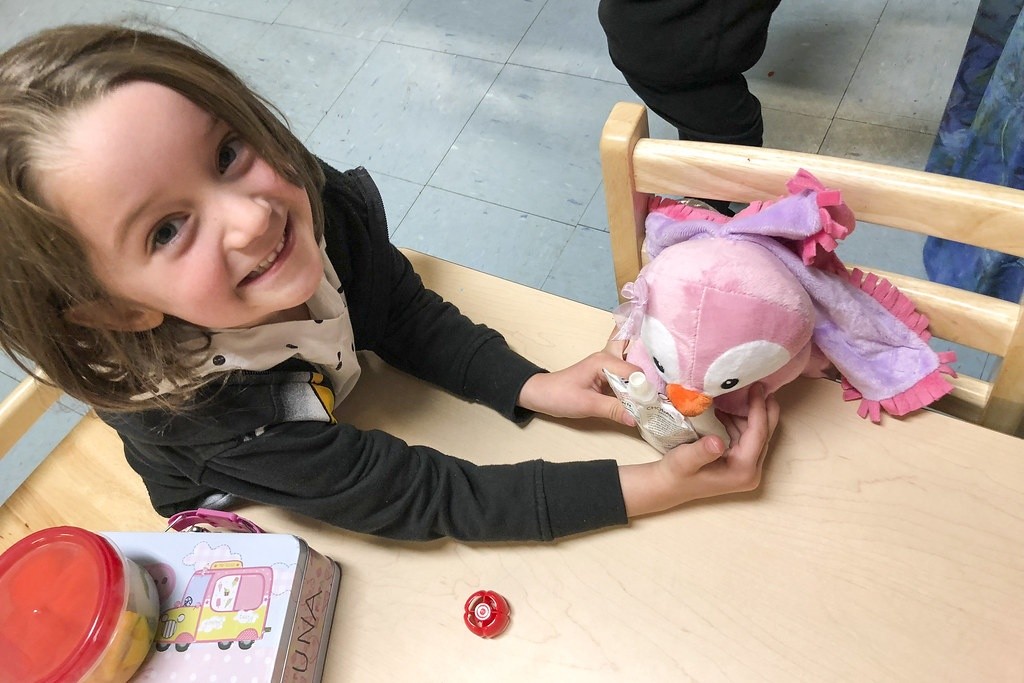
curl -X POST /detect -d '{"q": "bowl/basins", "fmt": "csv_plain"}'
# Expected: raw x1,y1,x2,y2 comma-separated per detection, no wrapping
0,525,159,683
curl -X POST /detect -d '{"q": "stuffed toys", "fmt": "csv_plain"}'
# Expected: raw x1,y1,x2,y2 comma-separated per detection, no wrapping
632,167,958,424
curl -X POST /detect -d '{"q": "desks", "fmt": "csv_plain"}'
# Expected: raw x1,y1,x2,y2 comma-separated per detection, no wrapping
0,246,1024,681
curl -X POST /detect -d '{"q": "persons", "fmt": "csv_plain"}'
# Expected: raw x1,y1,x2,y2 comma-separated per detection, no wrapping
1,26,781,542
598,0,780,218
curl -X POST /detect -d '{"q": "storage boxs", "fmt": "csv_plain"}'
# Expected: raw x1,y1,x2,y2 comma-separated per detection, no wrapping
93,532,344,683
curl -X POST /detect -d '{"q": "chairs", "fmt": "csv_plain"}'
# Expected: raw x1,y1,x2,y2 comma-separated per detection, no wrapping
599,101,1024,439
